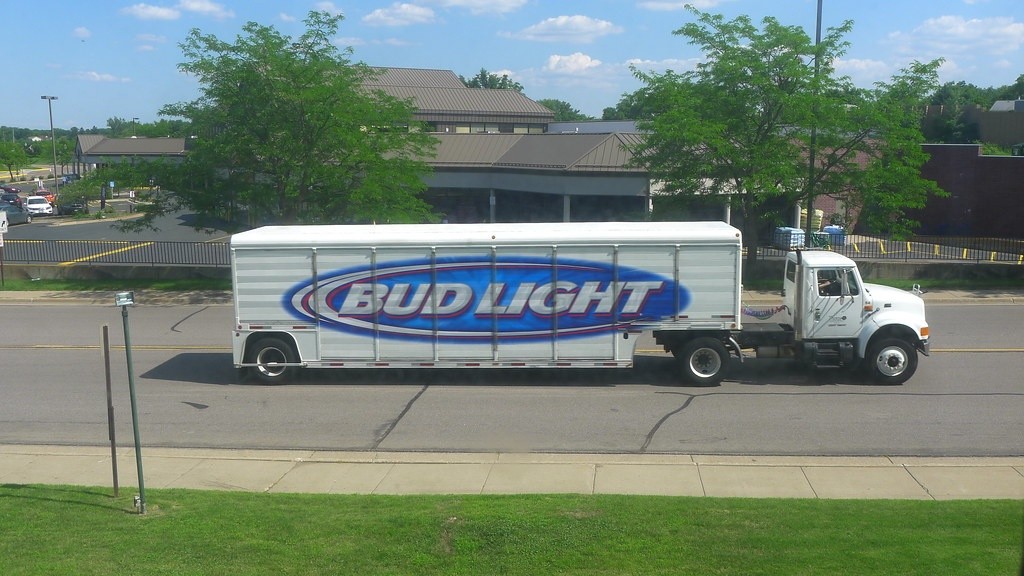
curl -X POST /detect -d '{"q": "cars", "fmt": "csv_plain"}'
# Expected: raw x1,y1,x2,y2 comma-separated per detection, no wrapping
63,174,80,185
0,184,86,227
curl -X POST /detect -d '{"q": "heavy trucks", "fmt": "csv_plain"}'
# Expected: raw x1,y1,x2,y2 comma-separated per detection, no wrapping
227,219,932,390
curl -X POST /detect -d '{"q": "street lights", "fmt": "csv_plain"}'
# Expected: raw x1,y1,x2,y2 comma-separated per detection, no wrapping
40,95,60,197
132,117,139,136
